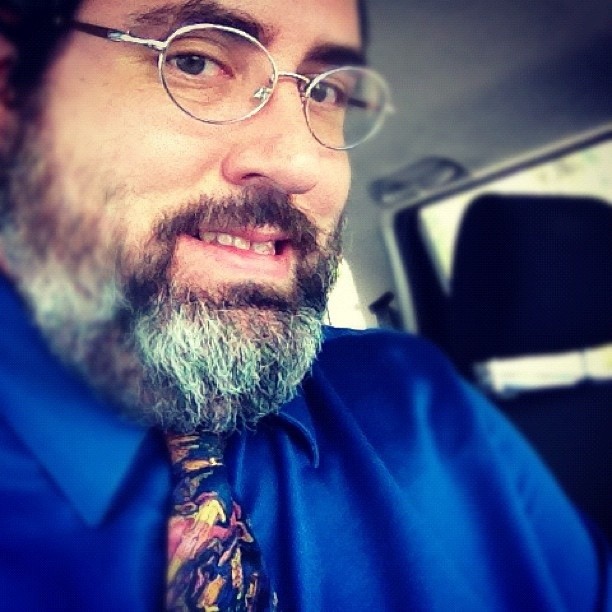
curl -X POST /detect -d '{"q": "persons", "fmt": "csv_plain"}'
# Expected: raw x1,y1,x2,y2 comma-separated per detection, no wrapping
1,1,611,611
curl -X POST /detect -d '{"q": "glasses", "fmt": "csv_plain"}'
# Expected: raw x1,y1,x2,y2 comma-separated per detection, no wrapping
68,23,396,151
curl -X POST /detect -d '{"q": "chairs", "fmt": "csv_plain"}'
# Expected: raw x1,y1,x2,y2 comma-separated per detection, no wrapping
447,191,612,555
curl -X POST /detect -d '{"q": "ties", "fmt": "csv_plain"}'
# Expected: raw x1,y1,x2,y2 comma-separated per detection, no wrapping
167,430,279,607
